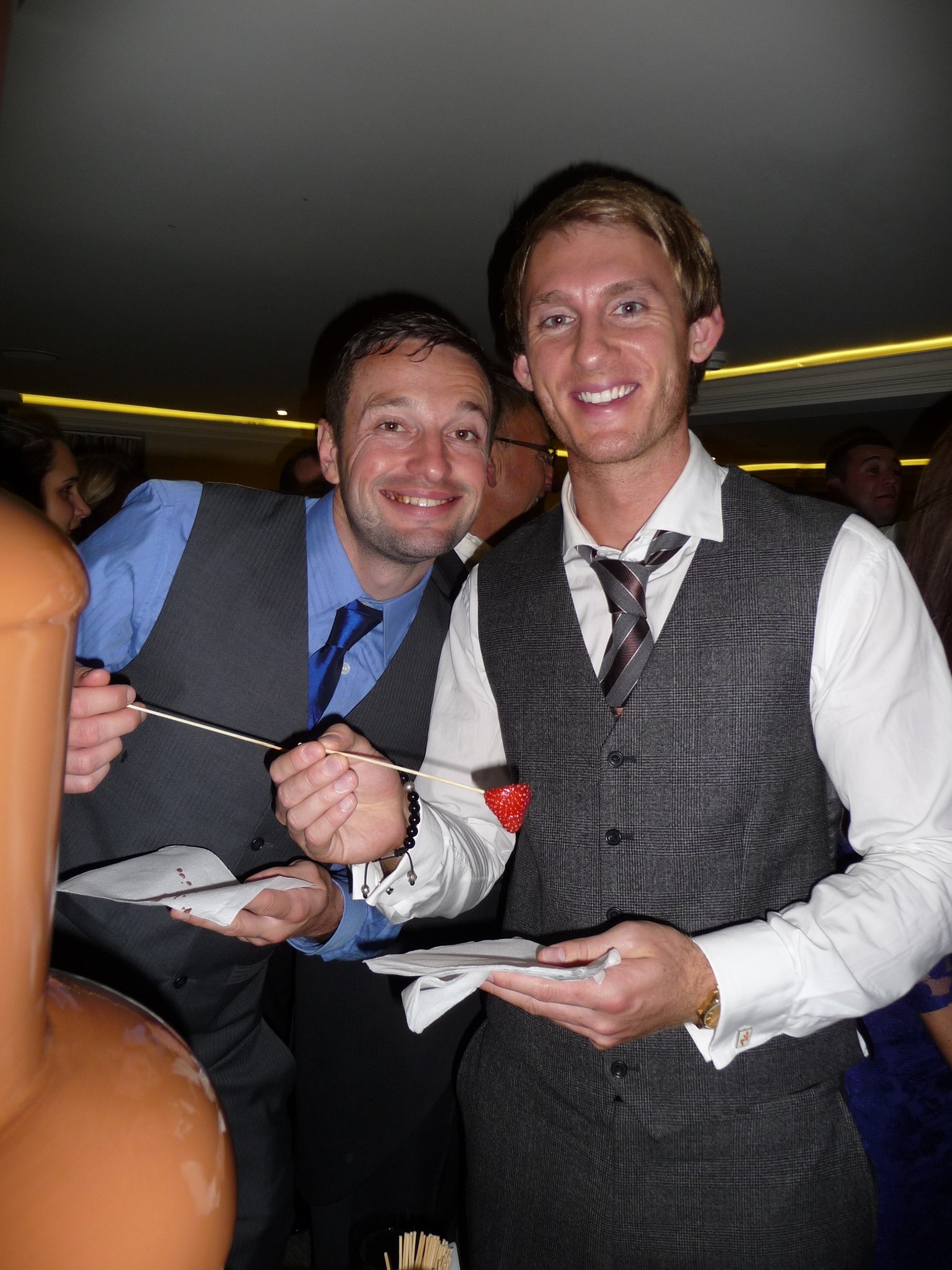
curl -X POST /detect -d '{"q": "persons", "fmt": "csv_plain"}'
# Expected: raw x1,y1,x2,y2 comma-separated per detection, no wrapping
62,290,504,1269
269,158,952,1269
0,386,565,1270
826,411,952,1270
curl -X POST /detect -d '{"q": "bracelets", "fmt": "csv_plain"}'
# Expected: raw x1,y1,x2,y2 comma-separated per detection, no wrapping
357,770,421,896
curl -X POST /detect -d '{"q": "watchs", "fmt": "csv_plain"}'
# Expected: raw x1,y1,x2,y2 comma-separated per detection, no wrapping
694,983,721,1029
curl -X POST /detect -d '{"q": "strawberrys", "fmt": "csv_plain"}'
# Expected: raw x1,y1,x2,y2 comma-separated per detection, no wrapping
485,785,531,833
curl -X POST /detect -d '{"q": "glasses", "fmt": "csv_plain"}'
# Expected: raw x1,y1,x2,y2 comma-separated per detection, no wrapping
492,436,557,467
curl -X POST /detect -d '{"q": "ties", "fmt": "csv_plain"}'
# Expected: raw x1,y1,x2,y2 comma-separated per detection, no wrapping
573,529,691,725
308,599,384,734
